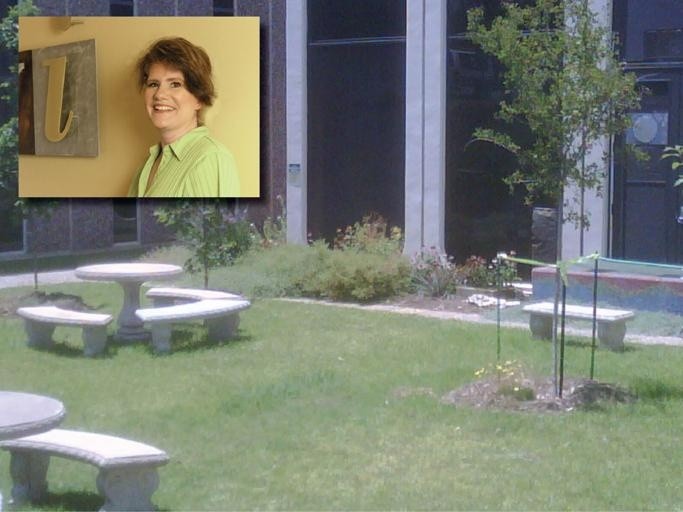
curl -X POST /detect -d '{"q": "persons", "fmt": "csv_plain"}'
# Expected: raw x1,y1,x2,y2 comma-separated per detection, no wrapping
126,35,244,198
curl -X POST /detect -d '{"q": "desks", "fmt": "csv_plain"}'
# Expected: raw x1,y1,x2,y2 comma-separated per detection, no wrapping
0,390,66,510
77,261,183,342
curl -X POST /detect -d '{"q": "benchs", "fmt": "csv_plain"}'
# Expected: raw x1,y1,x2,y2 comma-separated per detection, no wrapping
522,302,635,349
0,428,171,511
16,286,252,357
